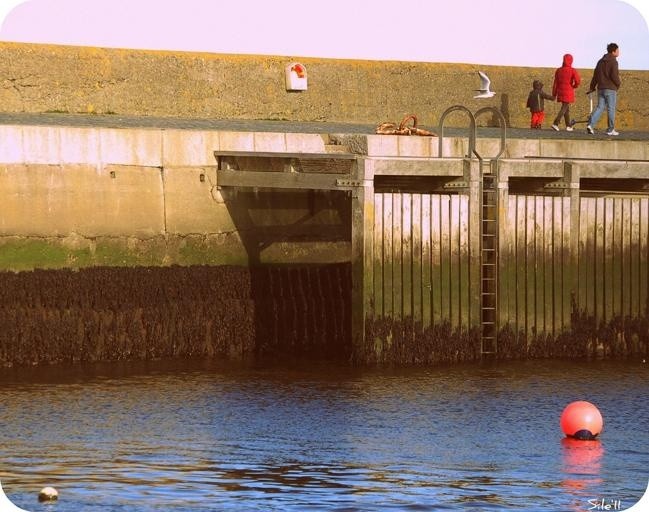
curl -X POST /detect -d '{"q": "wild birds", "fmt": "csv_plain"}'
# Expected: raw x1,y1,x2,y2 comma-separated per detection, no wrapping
472,71,497,100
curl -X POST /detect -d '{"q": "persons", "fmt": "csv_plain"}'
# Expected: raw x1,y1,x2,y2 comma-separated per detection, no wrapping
585,43,621,135
527,79,554,129
552,54,580,132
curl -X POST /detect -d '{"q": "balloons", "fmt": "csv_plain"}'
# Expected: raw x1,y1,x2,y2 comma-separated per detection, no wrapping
558,436,606,481
564,398,606,442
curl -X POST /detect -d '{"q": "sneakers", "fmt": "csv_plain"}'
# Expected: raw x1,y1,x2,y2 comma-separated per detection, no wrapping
551,124,559,131
605,129,619,135
566,124,575,131
586,124,594,134
